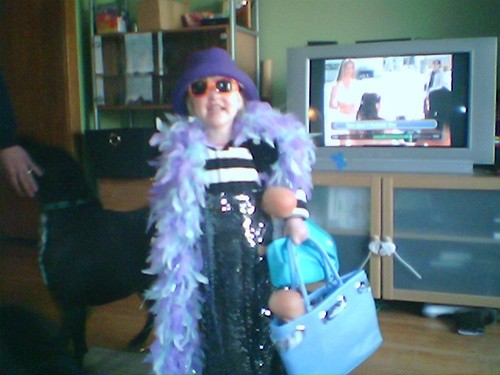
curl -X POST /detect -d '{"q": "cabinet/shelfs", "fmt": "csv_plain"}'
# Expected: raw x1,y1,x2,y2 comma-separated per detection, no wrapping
308,171,500,308
87,0,259,127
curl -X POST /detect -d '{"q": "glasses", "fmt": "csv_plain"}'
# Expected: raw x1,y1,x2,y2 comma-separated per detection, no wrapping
187,77,241,96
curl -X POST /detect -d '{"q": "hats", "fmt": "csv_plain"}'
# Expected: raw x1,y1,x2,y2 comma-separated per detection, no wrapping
172,46,260,115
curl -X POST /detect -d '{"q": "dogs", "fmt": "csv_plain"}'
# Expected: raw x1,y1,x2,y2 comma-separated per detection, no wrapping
18,135,157,364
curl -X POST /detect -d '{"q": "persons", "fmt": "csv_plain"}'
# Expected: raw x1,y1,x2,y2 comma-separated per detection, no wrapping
352,90,388,147
149,44,326,374
417,87,451,146
0,70,46,197
424,58,443,113
329,60,360,148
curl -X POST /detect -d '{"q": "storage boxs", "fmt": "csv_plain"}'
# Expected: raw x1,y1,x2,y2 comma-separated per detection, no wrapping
136,0,187,31
96,7,125,33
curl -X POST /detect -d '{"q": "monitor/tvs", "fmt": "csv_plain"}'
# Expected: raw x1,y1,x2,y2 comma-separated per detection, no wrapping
287,37,495,175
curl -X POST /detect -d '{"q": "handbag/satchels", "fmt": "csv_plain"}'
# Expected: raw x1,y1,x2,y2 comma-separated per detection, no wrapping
266,219,339,287
265,237,384,375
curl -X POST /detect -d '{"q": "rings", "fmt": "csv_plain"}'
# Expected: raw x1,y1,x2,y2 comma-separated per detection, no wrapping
26,168,35,175
